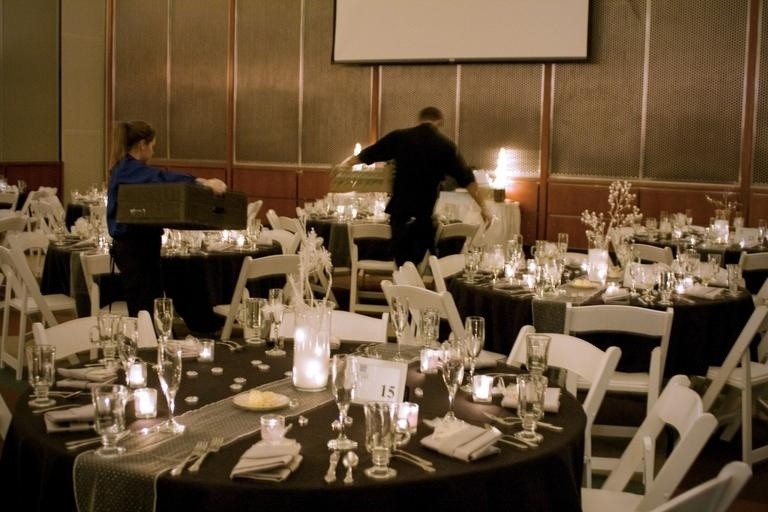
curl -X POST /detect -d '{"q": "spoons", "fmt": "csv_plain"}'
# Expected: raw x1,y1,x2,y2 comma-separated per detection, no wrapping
66,428,133,453
223,337,246,351
485,410,565,434
28,388,84,400
381,446,433,468
343,452,362,486
485,423,538,448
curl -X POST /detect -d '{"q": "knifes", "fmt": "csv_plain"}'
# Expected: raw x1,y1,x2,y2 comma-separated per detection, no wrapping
35,389,96,397
377,453,437,473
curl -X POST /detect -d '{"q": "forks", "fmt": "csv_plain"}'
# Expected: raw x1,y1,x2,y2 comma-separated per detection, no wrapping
169,437,222,481
325,449,340,486
217,341,239,352
66,427,133,449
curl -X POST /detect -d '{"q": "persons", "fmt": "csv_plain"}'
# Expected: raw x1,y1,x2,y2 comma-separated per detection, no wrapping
102,117,228,319
340,106,493,272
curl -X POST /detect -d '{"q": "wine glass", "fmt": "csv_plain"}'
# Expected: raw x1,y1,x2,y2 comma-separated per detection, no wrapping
55,181,109,252
237,290,287,364
326,296,557,454
165,219,264,256
461,233,576,296
628,206,767,308
154,298,174,357
154,344,188,434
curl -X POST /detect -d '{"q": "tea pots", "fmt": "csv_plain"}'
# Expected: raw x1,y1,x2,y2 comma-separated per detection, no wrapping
292,300,333,392
587,233,609,285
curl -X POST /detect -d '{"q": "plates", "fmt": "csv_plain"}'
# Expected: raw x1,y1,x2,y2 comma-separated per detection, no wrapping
233,390,292,413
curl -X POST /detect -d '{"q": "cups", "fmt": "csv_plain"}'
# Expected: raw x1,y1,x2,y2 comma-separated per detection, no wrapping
260,414,289,439
400,401,420,434
316,192,387,221
124,360,155,390
362,401,411,453
471,374,495,406
418,349,439,374
134,387,164,419
195,339,214,364
26,310,140,449
